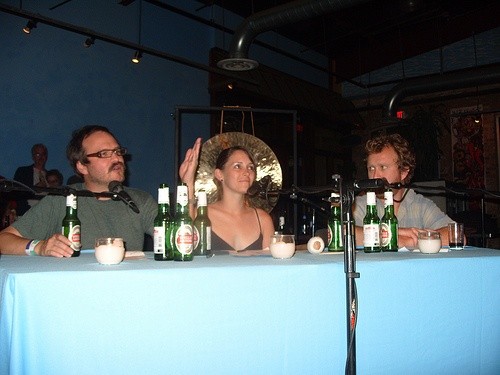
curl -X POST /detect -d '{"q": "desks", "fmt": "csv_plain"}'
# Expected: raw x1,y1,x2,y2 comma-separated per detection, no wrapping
0,247,500,375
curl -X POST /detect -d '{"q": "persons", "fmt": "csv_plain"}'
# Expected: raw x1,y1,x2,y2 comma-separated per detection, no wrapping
13,143,63,216
352,133,466,247
194,132,282,251
0,124,201,258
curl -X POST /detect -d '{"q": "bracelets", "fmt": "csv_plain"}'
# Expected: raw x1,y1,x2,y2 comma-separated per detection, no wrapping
25,239,41,256
189,199,197,205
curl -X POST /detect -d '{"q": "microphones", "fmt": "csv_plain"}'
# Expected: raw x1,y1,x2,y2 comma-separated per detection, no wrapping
108,181,140,213
345,177,383,190
247,175,270,196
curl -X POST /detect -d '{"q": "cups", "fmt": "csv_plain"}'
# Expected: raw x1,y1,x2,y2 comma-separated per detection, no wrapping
95,238,124,265
418,231,441,255
448,223,464,251
270,235,296,260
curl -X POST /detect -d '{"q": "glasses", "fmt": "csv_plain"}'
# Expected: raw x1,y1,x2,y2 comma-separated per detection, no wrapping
83,147,128,159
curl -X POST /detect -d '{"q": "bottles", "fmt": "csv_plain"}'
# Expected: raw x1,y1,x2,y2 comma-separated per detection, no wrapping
380,188,399,252
154,183,173,262
193,188,213,256
169,182,194,262
61,191,81,258
362,189,381,253
327,193,344,252
274,214,287,235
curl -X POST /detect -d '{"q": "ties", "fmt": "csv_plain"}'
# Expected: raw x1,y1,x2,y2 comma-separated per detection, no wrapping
39,172,47,186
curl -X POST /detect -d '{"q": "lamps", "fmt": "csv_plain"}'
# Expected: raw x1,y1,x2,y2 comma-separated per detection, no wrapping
23,19,39,34
131,49,143,63
83,36,97,48
